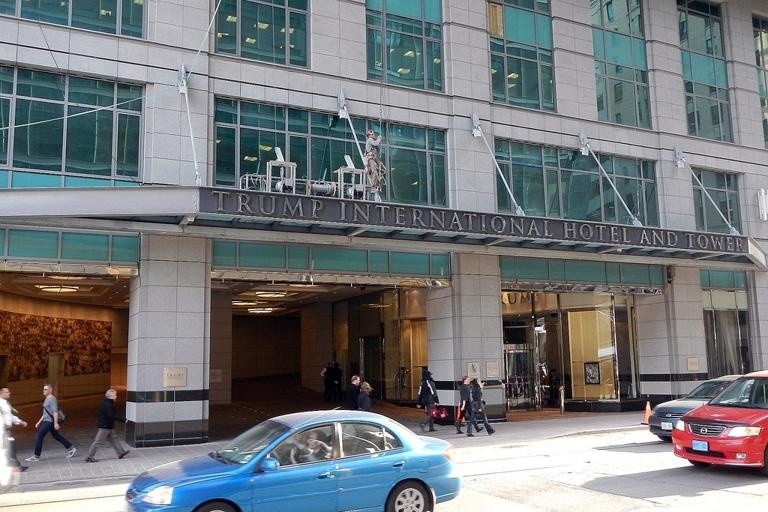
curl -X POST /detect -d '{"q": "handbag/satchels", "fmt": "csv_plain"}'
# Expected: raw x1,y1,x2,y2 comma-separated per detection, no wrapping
56,408,66,423
431,406,450,420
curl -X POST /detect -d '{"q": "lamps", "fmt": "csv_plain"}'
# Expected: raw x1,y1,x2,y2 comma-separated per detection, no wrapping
232,291,288,314
39,285,79,293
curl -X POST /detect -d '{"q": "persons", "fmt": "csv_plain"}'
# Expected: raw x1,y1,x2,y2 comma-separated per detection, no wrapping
319,361,334,401
328,362,344,403
364,129,383,194
0,385,29,473
83,387,130,463
356,380,375,412
465,377,496,437
453,375,483,434
342,374,361,410
415,369,440,432
305,429,329,462
24,382,77,462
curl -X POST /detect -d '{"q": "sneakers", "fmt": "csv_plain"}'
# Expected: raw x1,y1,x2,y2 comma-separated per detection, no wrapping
23,454,40,463
85,456,100,462
118,449,130,460
64,448,76,459
456,426,498,439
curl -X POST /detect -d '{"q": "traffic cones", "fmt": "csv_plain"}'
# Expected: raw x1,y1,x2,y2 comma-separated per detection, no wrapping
642,402,658,428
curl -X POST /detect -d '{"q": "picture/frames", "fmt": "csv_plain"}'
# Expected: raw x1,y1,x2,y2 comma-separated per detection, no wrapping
584,362,600,384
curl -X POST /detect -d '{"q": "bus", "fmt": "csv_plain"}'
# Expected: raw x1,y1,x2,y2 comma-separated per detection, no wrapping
125,407,463,512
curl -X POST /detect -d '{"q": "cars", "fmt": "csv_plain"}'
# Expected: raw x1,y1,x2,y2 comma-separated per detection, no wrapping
668,368,768,477
648,375,747,444
